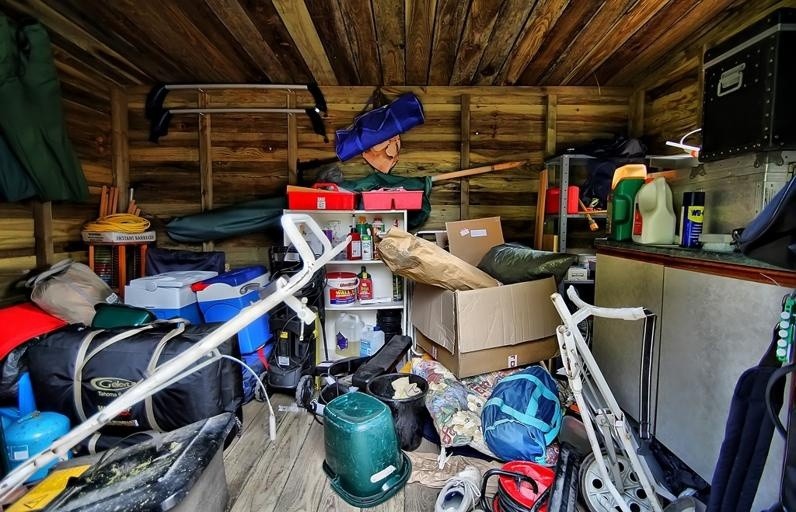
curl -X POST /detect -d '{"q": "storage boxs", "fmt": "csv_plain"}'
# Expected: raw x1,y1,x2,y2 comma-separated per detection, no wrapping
696,6,796,163
409,275,564,380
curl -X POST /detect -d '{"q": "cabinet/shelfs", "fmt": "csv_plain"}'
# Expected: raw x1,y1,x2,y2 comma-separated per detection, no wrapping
538,146,796,512
285,207,408,371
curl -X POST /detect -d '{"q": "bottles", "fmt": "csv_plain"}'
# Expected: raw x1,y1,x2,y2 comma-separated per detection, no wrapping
356,265,372,301
346,214,385,261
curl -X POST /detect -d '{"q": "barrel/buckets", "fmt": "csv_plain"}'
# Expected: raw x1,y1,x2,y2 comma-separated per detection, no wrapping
321,270,360,309
355,217,375,261
360,323,386,360
607,176,647,239
629,177,677,247
321,389,412,506
334,312,362,359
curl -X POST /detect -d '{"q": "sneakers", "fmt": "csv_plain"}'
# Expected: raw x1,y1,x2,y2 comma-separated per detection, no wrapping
433,465,482,512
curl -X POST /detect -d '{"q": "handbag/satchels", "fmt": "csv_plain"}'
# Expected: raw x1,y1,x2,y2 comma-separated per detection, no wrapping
25,321,243,454
332,85,427,163
480,366,562,467
25,257,120,331
731,172,795,271
